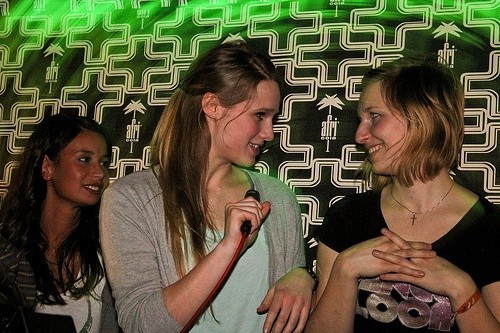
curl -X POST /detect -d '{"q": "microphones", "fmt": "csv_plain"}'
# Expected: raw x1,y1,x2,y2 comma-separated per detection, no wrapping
241,190,261,234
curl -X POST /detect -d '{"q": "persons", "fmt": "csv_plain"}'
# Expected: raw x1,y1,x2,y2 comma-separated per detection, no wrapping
303,57,500,333
0,113,120,333
99,42,314,333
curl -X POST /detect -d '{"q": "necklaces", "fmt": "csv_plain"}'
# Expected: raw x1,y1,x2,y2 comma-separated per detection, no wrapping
390,182,454,225
79,298,93,333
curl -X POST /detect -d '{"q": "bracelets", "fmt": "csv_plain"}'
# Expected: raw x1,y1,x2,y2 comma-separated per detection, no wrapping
450,290,482,326
299,267,319,292
